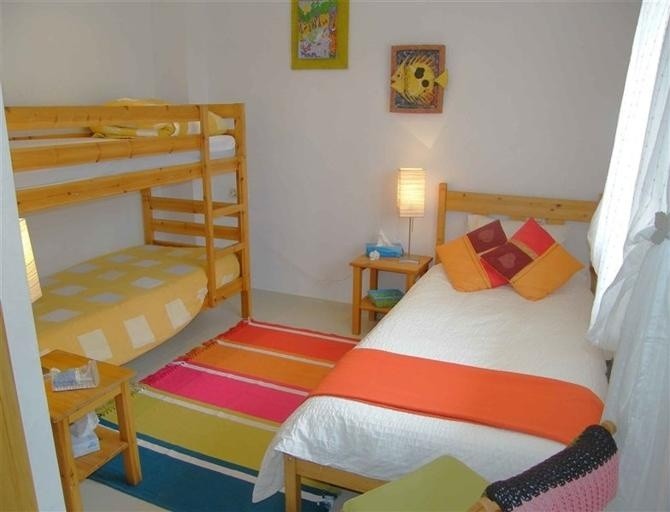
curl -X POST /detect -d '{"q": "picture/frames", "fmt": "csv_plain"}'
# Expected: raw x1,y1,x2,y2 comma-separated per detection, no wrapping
288,0,349,70
390,44,448,114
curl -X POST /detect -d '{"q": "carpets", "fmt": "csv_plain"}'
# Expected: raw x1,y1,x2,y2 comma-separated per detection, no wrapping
74,315,360,512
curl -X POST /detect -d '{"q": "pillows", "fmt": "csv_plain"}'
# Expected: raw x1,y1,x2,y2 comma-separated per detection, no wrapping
434,218,585,302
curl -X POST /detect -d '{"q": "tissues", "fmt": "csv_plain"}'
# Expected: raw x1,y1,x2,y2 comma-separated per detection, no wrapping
365,232,402,258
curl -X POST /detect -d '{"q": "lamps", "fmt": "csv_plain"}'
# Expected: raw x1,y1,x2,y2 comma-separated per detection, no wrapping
394,166,426,267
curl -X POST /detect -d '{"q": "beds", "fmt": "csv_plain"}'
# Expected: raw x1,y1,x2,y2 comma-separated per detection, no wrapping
1,99,248,384
281,181,615,510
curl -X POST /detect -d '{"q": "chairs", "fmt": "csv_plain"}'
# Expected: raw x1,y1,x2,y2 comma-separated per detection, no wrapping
345,420,618,511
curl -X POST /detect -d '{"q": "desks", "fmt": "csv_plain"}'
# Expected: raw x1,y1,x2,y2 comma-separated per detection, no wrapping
33,348,145,512
351,250,431,335
